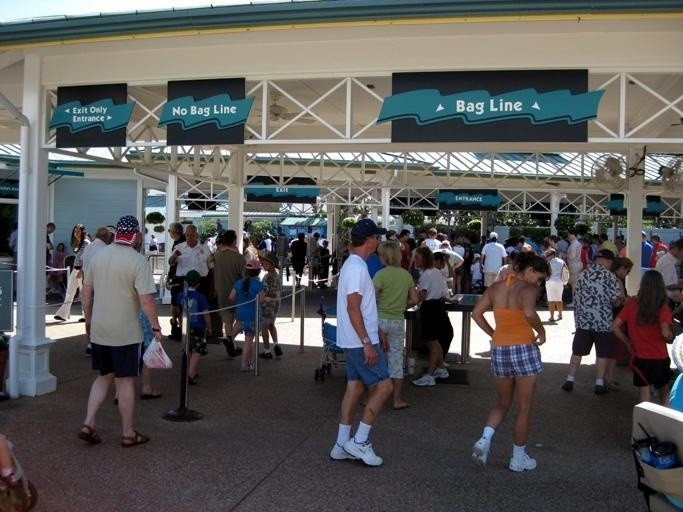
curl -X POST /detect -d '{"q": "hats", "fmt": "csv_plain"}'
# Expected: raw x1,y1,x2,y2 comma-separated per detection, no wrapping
258,253,281,269
351,219,387,239
490,232,498,239
114,215,139,245
246,260,262,269
594,249,616,260
441,240,450,246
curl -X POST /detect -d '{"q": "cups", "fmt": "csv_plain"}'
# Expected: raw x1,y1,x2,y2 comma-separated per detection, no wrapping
630,436,678,468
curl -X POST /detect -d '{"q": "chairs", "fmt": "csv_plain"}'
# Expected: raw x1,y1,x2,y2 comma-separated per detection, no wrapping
625,401,682,512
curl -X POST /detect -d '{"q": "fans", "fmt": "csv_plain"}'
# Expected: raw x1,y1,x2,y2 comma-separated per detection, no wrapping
591,151,631,195
655,151,682,192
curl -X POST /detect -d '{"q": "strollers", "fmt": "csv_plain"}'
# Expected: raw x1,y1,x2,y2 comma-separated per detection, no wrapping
310,295,349,383
0,476,40,512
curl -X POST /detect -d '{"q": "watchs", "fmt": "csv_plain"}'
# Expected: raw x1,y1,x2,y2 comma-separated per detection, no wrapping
360,337,370,344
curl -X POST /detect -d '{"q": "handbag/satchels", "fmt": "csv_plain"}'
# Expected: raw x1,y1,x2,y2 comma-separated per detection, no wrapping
670,304,683,336
561,264,570,285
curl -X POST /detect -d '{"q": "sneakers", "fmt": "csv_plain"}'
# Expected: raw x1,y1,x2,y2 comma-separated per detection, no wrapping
508,453,538,473
188,374,199,385
470,438,490,472
274,344,283,357
593,384,606,395
431,367,450,378
329,437,384,468
412,374,436,387
561,381,574,392
259,351,273,359
83,347,94,357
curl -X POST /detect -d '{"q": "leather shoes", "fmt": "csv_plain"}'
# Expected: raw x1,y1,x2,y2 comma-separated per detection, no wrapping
54,316,66,321
79,319,86,322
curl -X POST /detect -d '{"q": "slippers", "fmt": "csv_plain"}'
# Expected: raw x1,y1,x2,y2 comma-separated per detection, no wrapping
393,403,411,410
139,387,162,400
360,399,368,407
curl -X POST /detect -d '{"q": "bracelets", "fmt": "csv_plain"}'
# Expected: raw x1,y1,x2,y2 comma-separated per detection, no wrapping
152,327,159,331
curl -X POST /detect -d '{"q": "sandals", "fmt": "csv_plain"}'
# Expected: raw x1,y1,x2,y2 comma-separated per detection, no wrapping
239,359,256,371
77,425,101,444
222,336,243,357
120,429,150,447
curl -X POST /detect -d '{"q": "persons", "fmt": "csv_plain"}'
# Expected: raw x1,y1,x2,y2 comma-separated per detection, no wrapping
0,437,21,488
659,331,682,509
329,219,393,466
470,251,547,472
75,215,161,446
